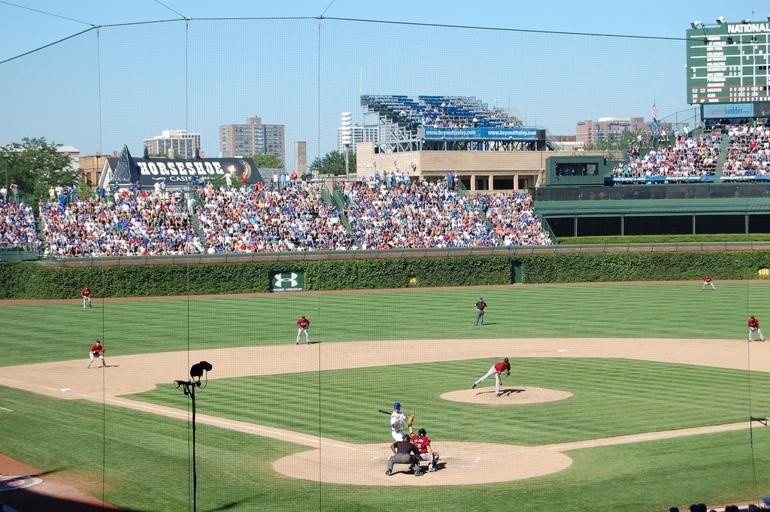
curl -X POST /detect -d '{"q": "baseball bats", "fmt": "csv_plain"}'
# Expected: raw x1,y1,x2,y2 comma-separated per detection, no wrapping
378,409,391,414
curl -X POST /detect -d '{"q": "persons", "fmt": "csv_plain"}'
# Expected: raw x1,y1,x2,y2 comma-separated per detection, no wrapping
703,275,716,290
385,434,424,476
475,298,487,325
472,358,510,397
748,315,764,342
408,416,439,472
87,342,105,368
391,402,407,454
296,315,311,344
81,287,91,308
669,497,770,512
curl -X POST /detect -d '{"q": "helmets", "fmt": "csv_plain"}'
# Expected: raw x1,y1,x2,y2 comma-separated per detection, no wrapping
402,435,412,440
504,358,509,362
393,402,401,409
418,428,426,435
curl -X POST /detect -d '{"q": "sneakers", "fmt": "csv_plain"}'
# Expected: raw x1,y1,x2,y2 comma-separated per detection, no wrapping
472,384,475,388
414,470,423,475
385,470,392,474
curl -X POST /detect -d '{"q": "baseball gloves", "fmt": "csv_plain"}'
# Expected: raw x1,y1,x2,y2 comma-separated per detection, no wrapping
407,415,415,427
